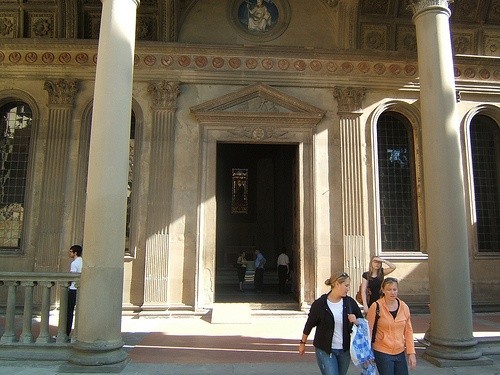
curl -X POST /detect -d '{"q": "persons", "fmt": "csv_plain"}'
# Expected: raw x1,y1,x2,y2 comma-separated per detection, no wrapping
299,273,364,375
365,277,416,375
254,249,267,293
277,248,290,291
236,251,247,291
52,245,82,341
361,256,396,318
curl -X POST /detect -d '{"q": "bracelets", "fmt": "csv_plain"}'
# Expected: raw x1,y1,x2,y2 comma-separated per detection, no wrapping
300,340,306,345
384,260,385,262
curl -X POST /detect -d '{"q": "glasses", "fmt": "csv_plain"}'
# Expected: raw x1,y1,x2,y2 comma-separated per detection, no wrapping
69,251,73,253
331,273,347,284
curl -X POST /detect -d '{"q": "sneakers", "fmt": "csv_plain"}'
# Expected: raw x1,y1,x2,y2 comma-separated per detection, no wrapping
52,335,69,340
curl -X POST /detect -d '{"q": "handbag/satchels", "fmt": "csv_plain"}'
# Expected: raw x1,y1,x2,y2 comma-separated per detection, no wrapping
371,301,380,343
350,318,375,367
356,284,368,306
